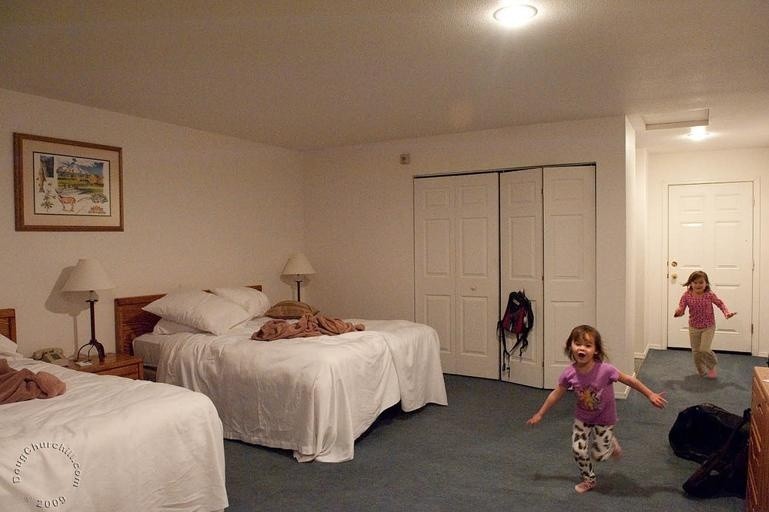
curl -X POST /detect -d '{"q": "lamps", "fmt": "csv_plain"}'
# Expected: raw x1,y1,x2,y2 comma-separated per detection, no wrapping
62,258,114,361
281,254,315,300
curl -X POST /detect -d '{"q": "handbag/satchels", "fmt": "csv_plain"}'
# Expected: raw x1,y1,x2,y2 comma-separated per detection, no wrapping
668,401,750,499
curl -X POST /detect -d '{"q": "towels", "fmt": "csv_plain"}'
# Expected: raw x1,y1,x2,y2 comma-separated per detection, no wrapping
251,315,366,341
1,358,67,406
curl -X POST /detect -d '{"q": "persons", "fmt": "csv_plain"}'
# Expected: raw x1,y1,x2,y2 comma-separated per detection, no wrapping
526,325,668,493
673,271,736,379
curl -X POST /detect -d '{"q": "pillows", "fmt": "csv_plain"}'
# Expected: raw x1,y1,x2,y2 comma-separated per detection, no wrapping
207,284,272,317
153,317,195,335
0,333,18,354
141,283,259,336
264,298,320,319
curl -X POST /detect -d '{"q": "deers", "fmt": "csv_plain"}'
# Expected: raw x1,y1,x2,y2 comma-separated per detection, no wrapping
54,188,77,211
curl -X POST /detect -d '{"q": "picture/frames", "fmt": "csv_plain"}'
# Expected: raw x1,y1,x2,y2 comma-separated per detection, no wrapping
12,131,124,232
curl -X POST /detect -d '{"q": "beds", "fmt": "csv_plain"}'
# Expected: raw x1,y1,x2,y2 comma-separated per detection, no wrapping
113,285,449,464
0,308,230,512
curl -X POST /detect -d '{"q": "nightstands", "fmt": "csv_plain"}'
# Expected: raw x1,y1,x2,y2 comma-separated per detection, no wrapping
66,353,145,381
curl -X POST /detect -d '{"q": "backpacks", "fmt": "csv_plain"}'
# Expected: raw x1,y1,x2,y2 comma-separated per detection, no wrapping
497,291,533,377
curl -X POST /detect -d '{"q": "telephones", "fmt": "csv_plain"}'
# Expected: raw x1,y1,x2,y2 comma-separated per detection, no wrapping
32,347,69,367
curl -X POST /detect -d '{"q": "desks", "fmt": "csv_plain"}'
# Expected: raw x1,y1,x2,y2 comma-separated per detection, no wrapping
746,365,768,511
